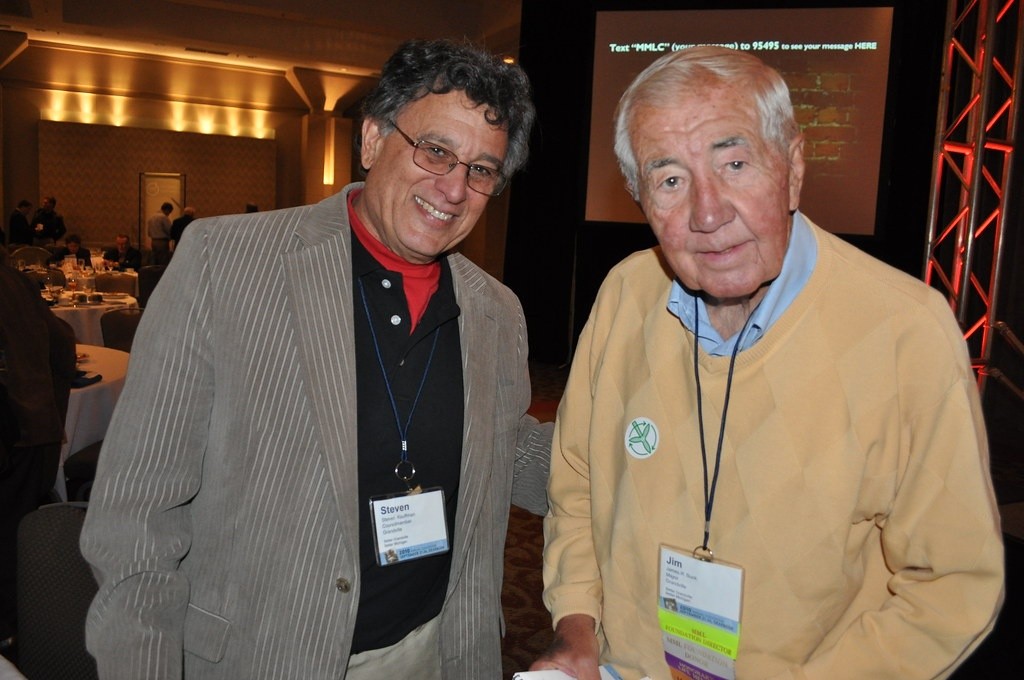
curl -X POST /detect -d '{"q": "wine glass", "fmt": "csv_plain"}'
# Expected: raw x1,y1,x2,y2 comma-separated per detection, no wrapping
67,276,79,294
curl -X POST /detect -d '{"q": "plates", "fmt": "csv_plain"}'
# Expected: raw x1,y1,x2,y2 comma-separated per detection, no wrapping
77,352,90,361
97,291,128,298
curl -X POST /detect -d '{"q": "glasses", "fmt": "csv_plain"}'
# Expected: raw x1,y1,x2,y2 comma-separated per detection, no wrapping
387,117,509,198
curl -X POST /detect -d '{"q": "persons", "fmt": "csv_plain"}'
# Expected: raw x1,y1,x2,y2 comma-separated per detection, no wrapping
170,206,197,252
79,38,557,680
0,194,69,251
527,46,1006,680
101,231,143,274
0,260,80,669
52,233,93,271
147,202,174,266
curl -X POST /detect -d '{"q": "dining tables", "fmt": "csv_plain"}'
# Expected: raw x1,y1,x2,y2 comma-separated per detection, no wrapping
49,342,129,501
59,269,139,297
41,290,139,347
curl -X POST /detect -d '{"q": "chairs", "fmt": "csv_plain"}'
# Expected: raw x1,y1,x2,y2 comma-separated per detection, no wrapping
0,233,163,680
